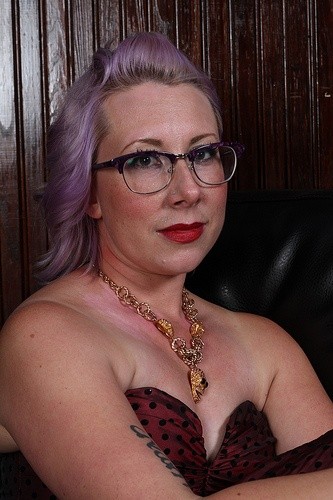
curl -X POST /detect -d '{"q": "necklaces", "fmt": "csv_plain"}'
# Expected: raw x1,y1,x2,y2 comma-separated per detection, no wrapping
91,264,209,404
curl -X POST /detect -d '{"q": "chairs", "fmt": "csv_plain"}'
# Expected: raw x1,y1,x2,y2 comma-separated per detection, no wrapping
185,190,333,402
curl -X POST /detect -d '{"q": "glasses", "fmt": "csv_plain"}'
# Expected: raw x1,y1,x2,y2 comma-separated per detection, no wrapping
92,141,242,192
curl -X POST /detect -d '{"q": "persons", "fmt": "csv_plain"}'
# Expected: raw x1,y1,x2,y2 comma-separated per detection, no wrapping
0,34,333,500
